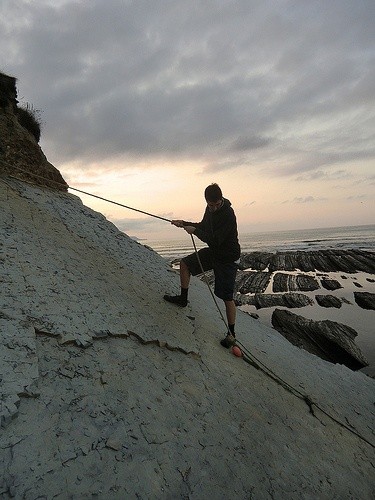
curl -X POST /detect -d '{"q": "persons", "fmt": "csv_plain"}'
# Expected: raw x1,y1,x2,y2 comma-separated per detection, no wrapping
161,183,241,349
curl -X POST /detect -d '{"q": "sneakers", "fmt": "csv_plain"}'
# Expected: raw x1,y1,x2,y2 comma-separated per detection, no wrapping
221,336,235,348
164,294,187,307
